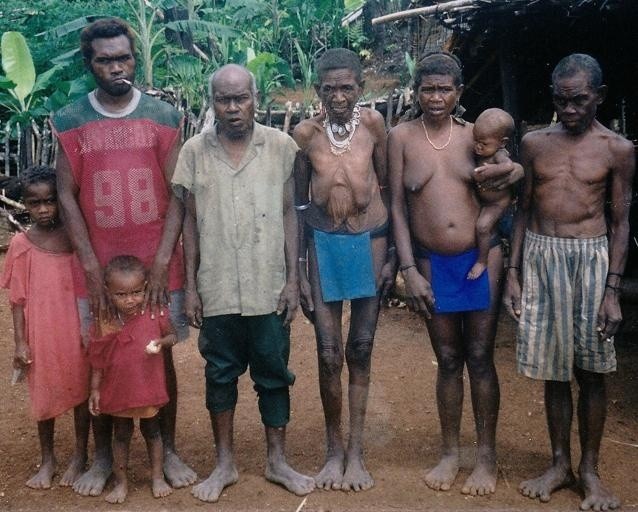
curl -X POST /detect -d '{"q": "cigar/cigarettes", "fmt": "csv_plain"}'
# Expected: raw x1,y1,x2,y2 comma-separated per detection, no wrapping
600,333,612,344
121,78,134,86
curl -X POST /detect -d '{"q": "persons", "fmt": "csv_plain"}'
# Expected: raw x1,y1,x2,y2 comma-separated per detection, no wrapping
48,14,198,497
168,62,318,502
85,255,180,504
498,52,635,512
1,164,92,490
291,46,393,494
462,103,515,282
385,51,526,498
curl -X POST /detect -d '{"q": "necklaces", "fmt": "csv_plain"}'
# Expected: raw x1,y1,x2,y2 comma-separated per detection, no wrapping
419,112,454,152
321,105,363,157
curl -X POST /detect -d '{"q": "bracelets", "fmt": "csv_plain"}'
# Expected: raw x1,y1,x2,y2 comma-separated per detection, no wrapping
507,265,521,272
603,270,625,281
299,256,308,264
399,262,418,273
605,282,627,294
